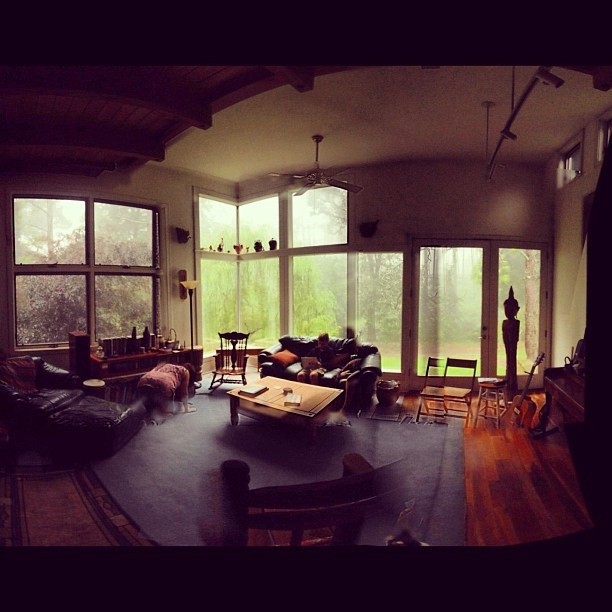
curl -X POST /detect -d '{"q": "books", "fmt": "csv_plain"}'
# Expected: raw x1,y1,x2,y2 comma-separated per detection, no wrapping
238,384,269,398
284,393,302,407
301,357,320,370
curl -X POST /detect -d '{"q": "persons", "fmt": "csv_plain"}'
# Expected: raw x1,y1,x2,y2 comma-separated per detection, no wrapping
136,363,196,426
297,333,329,385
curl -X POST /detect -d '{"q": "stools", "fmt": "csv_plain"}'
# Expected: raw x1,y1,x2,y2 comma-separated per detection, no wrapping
472,380,509,430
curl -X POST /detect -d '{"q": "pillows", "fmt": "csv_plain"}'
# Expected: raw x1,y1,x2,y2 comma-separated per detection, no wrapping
342,357,362,372
267,348,299,368
334,352,351,366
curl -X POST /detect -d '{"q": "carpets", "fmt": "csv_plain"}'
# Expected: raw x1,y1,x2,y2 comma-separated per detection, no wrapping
89,363,468,546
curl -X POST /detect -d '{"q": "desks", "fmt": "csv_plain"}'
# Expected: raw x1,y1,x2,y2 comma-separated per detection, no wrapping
529,366,585,438
213,348,264,372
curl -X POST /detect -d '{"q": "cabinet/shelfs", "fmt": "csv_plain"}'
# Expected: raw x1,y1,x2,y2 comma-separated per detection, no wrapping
85,347,193,407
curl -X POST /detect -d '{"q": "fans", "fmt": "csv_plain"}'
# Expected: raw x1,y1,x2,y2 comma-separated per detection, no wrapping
267,134,364,197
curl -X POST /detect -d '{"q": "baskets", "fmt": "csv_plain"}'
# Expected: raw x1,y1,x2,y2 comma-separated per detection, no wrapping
377,380,401,405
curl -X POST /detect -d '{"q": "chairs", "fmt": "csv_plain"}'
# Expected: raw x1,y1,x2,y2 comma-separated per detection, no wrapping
416,357,448,423
443,358,477,429
208,331,251,390
220,450,428,546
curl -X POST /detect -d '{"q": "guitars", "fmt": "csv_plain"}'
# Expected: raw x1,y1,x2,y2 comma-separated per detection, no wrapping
501,351,545,444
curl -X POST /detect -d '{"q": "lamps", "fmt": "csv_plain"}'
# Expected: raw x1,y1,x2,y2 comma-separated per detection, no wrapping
180,278,203,390
499,128,517,142
532,69,564,89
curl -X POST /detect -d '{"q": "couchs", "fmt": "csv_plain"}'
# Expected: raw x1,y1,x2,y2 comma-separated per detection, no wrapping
257,334,382,413
0,353,143,459
